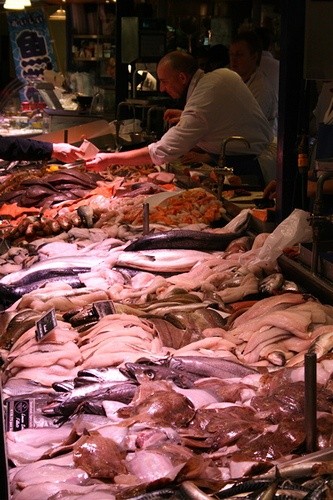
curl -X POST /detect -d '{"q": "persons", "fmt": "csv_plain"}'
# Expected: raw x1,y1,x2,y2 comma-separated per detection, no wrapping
0,135,86,165
200,0,294,164
74,53,274,191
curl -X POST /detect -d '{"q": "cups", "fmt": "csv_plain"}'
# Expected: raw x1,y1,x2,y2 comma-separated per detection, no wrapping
41,116,51,134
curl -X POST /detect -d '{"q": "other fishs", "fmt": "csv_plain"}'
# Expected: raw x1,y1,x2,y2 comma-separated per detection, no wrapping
0,164,333,500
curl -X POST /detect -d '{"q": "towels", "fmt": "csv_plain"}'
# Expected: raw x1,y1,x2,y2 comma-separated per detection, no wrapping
311,81,333,125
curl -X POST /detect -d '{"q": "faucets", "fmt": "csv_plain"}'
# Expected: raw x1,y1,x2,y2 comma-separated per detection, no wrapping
220,135,251,159
